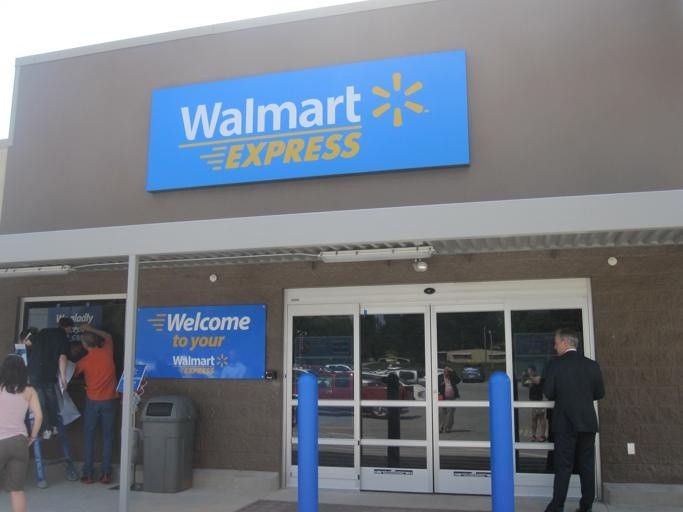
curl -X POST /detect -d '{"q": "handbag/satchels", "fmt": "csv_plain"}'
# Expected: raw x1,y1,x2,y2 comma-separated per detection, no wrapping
62,388,82,426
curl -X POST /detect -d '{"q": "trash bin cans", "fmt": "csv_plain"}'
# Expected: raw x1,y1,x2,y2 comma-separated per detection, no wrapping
139,396,198,493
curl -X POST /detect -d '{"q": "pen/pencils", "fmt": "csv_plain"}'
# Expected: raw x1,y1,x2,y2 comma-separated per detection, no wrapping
22,332,32,344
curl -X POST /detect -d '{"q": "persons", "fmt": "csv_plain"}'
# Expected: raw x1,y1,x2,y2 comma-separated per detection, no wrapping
541,325,604,512
522,366,547,443
0,317,120,512
438,366,460,434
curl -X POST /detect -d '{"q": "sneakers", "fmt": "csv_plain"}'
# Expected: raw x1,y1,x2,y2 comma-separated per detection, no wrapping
79,476,94,484
99,472,112,483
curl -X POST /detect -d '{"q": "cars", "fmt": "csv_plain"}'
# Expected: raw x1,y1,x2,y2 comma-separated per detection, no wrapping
461,365,484,383
292,356,447,420
